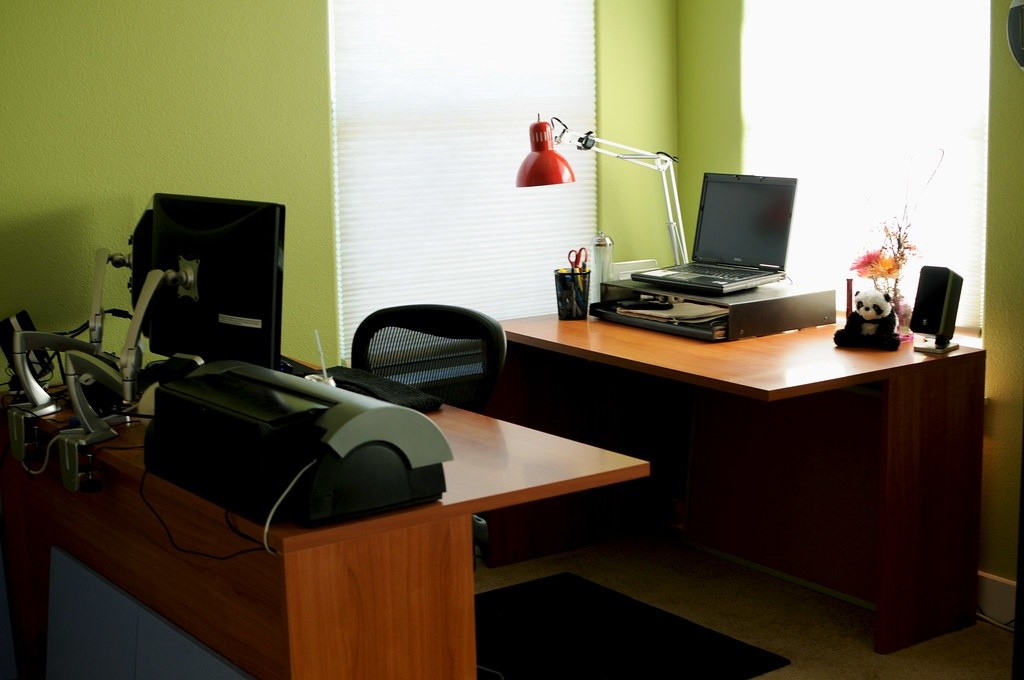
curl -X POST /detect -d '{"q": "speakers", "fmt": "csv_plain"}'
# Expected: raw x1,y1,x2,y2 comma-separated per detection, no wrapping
910,266,963,353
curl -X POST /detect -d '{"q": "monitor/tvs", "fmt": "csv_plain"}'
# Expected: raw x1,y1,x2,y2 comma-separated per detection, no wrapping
128,192,287,367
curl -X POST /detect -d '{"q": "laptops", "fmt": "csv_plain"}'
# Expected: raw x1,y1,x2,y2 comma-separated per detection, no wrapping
631,173,798,294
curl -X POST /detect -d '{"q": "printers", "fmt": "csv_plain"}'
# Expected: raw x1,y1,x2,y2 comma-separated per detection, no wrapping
142,361,456,533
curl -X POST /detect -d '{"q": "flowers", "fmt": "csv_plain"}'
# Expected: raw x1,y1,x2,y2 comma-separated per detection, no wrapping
850,147,945,333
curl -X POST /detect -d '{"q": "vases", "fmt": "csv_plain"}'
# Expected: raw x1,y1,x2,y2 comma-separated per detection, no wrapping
891,297,914,342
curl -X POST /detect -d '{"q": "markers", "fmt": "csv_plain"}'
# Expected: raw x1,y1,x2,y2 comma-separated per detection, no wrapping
558,263,589,315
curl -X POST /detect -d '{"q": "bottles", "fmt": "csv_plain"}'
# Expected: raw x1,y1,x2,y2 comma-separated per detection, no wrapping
592,231,614,303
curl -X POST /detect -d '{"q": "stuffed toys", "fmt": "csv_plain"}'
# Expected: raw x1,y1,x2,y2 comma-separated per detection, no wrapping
834,288,901,352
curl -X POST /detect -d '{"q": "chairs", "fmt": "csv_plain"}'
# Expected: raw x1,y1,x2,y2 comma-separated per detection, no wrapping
352,304,508,573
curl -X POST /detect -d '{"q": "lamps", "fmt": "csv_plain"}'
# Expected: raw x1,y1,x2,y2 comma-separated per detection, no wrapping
515,112,690,267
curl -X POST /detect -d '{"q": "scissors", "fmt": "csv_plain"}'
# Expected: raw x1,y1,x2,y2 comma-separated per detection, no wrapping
568,247,588,273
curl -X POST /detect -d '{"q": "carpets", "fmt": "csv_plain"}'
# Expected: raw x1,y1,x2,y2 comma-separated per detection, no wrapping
474,572,791,680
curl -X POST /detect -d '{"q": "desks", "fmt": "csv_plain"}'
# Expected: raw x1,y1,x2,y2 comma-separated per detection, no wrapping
0,358,650,680
495,311,987,652
